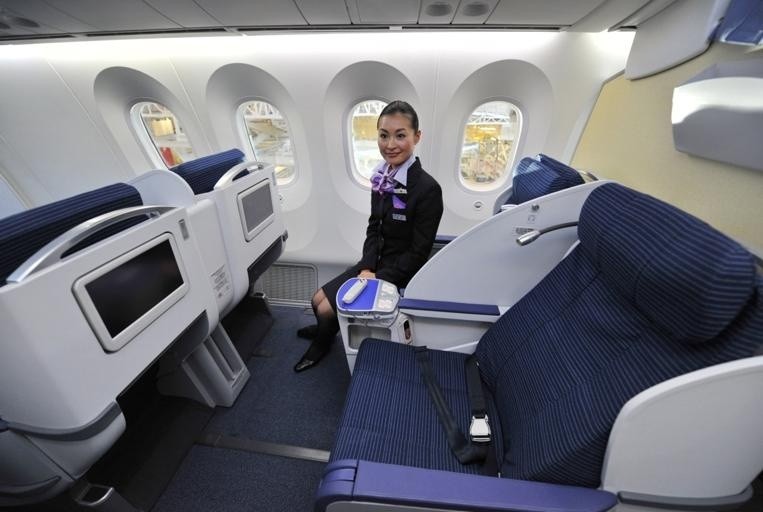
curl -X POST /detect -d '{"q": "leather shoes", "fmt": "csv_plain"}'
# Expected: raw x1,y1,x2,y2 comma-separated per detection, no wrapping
296,325,319,340
294,340,333,372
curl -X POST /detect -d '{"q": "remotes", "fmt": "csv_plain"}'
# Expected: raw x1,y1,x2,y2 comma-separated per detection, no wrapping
342,278,368,304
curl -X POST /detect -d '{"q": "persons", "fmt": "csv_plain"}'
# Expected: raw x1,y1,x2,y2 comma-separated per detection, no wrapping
292,100,444,374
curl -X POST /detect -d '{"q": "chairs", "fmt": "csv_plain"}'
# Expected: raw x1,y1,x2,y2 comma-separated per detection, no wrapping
317,152,763,512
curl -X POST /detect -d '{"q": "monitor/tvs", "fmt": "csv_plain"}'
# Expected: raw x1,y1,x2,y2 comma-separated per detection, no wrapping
237,178,276,242
73,232,191,352
